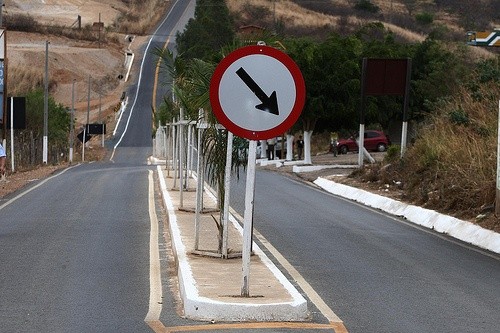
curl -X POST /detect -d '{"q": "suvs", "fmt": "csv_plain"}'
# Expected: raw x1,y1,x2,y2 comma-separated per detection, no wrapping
243,134,296,159
330,128,391,155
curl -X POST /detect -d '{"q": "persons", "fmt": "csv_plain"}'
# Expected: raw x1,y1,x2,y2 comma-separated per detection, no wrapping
0,143,7,173
267,137,277,160
296,135,304,160
